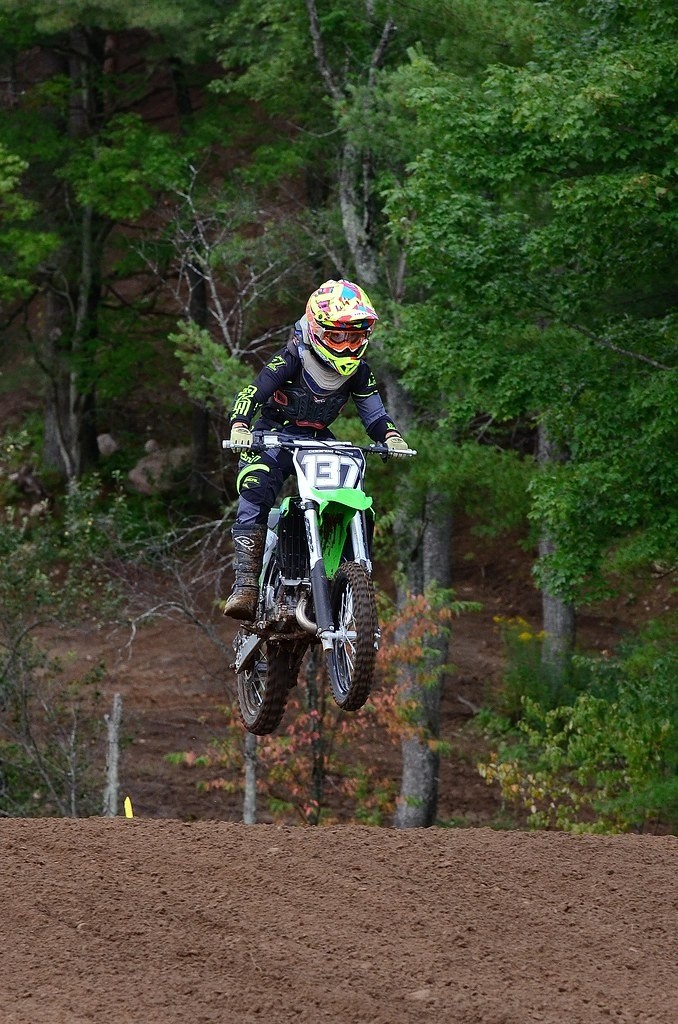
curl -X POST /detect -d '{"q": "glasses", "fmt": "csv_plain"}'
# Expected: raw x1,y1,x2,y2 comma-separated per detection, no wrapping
320,330,366,352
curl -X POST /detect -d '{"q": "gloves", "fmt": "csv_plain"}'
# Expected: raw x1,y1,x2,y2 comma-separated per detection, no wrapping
231,424,253,453
381,435,408,463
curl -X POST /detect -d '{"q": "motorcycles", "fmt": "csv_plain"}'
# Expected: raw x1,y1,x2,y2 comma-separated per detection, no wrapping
222,429,418,736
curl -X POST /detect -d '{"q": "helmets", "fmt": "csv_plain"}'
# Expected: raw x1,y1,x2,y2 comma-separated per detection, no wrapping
306,279,379,376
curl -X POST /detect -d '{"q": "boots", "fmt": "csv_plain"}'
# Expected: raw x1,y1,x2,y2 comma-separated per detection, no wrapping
224,523,267,620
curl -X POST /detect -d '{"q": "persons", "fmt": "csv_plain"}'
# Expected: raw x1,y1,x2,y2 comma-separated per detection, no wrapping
222,280,410,621
